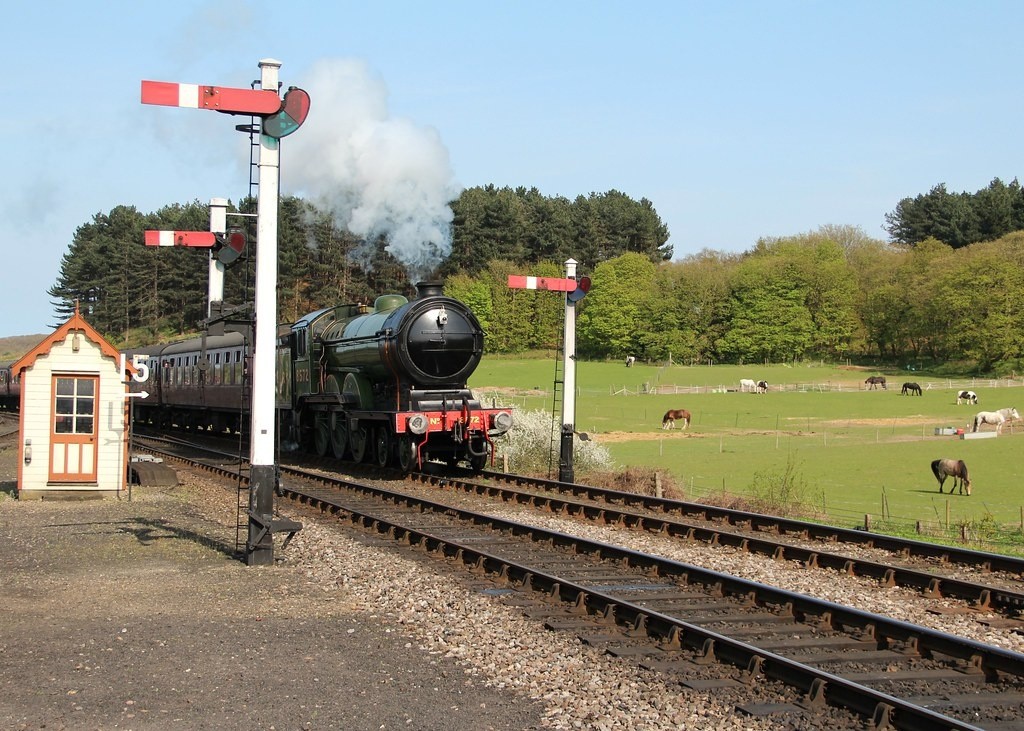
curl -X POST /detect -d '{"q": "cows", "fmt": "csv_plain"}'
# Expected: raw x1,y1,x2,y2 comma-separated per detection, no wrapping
662,409,691,430
740,379,768,393
901,382,923,396
626,356,635,367
865,376,887,390
956,391,978,405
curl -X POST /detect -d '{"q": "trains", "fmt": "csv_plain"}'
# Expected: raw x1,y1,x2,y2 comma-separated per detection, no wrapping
0,277,516,475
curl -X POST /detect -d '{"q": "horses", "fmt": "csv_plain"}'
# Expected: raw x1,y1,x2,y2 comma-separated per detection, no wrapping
973,406,1019,434
931,458,971,495
